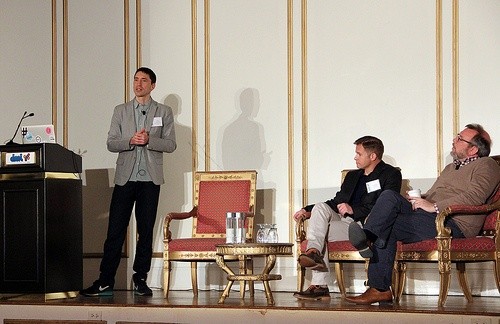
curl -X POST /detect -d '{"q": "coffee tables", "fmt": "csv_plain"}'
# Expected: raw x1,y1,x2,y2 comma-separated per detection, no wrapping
215,243,294,306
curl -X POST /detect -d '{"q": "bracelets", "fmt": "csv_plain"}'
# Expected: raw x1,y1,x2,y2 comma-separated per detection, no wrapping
433,202,440,214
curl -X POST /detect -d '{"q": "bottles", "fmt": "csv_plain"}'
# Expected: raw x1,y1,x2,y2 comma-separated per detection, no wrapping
226,212,237,243
236,212,246,243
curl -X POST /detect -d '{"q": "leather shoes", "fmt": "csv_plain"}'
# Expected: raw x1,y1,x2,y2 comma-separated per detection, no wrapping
348,221,375,258
344,287,394,305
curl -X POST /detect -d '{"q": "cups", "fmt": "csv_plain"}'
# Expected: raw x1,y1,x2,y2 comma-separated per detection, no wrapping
408,189,421,206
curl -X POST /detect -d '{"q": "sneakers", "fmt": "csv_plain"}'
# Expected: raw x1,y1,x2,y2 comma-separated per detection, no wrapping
134,279,153,297
79,280,114,296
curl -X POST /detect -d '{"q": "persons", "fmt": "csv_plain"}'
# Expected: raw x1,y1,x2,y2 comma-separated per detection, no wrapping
343,123,500,307
292,135,403,301
78,67,178,297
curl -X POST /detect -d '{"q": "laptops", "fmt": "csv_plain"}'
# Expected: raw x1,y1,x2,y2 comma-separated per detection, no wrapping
20,125,56,144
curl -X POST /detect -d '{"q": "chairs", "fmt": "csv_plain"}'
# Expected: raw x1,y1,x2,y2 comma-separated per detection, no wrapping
163,170,258,299
296,156,500,306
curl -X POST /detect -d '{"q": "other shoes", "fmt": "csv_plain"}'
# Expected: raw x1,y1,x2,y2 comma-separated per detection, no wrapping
293,286,332,301
298,253,326,271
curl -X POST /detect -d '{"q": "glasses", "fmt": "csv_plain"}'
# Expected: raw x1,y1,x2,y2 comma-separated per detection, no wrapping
455,133,474,146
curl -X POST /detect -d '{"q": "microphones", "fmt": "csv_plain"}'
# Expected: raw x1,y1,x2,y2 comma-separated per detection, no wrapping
6,111,34,145
142,111,146,115
455,165,459,170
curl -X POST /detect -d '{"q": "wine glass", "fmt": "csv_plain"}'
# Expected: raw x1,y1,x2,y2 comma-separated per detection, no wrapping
268,224,278,243
256,223,268,243
